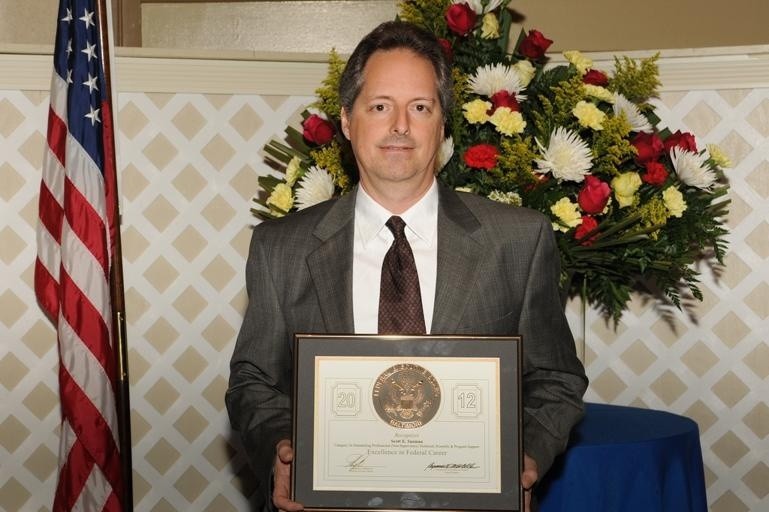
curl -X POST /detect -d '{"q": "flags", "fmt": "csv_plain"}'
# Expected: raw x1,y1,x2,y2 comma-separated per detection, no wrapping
34,0,124,512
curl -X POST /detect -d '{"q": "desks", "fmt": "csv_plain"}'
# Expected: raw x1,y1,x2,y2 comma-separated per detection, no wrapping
533,398,711,512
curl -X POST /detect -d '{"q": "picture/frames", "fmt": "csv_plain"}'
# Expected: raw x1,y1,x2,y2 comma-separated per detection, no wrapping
283,328,530,510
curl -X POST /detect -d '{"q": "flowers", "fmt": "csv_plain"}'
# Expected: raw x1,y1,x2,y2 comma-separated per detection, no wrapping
243,1,741,338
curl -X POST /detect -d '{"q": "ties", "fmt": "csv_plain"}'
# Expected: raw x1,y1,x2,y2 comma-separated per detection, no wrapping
378,215,427,336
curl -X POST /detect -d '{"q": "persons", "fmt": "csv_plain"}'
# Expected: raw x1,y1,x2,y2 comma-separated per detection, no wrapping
225,23,589,512
